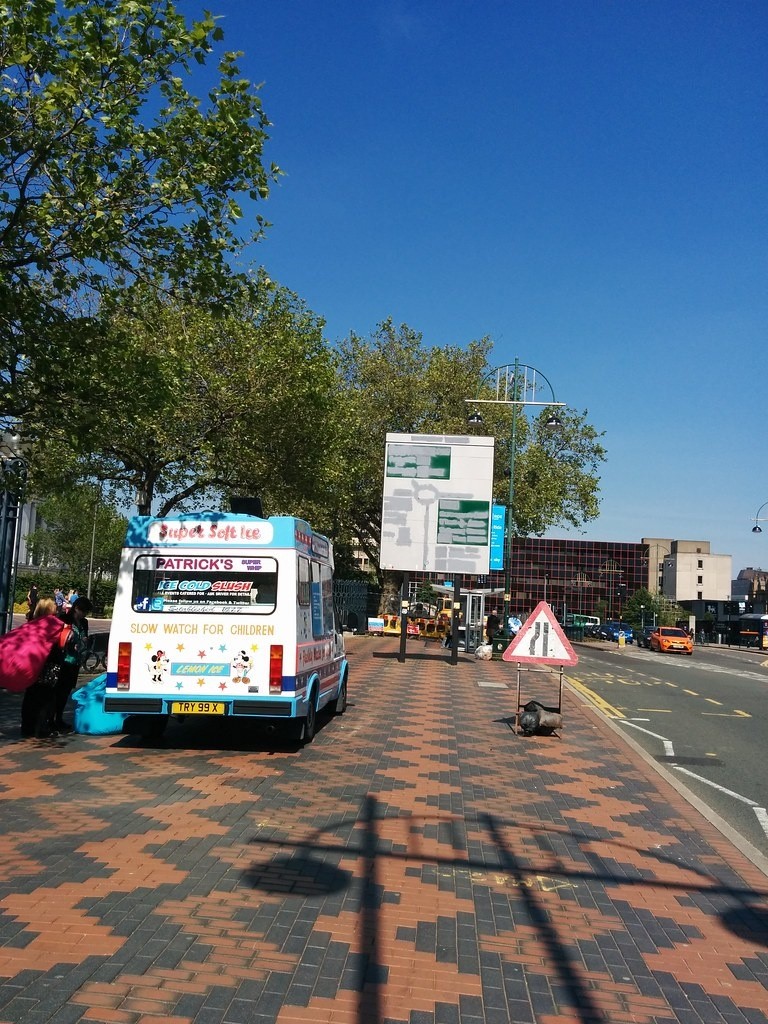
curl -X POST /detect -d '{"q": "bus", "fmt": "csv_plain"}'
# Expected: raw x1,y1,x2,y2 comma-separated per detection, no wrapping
561,613,601,626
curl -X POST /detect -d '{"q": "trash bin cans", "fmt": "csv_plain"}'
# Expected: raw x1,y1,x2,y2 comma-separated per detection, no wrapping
490,627,514,659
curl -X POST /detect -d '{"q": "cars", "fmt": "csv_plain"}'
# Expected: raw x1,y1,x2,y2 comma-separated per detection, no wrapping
639,626,695,655
583,622,636,645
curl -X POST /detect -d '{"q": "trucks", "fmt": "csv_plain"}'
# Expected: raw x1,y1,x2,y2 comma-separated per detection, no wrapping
101,510,350,749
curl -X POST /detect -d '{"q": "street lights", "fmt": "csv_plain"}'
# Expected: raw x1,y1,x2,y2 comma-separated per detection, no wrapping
598,558,624,621
465,356,568,652
642,543,674,626
570,571,594,614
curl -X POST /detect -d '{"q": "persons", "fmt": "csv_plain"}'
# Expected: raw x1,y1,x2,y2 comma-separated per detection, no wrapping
486,610,502,645
688,627,695,644
700,628,706,645
17,596,73,737
25,584,79,619
48,596,92,736
